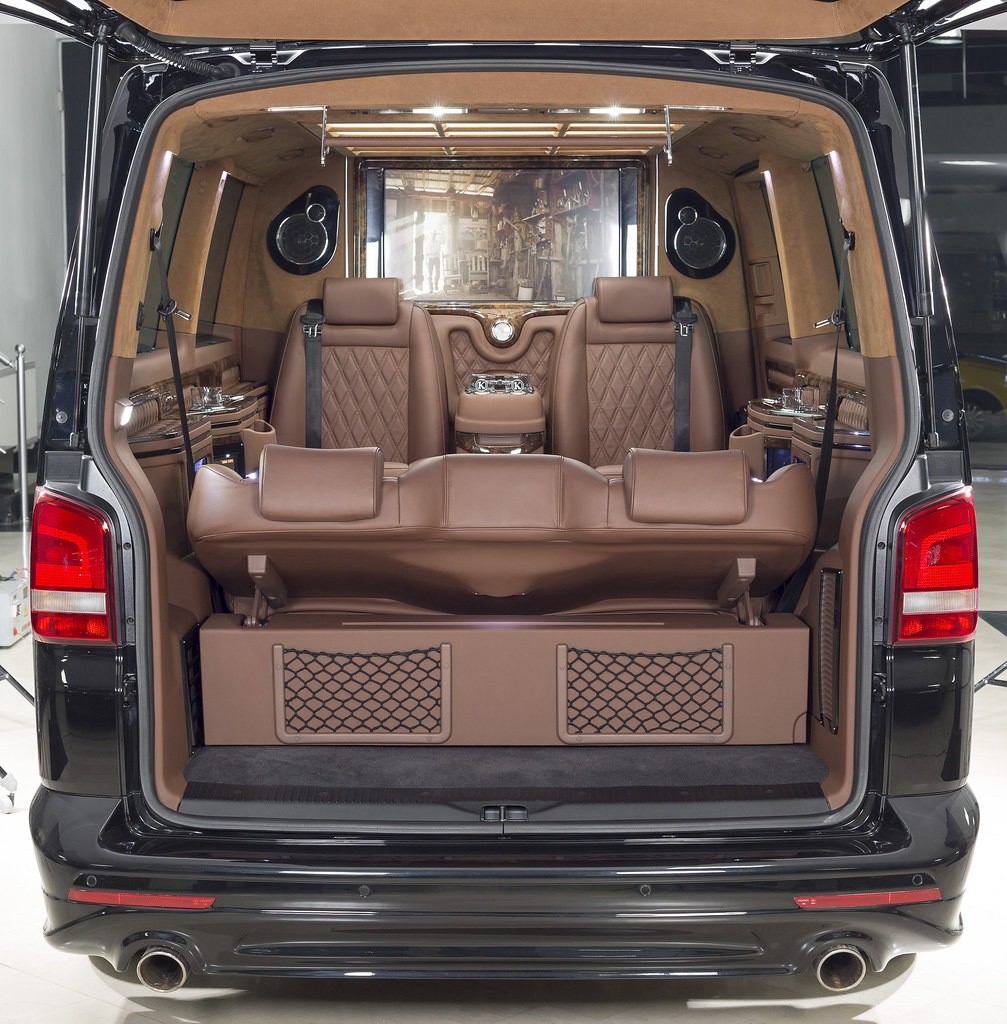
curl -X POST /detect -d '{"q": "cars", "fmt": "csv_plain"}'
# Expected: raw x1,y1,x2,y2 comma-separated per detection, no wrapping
1,0,1006,1003
954,347,1007,439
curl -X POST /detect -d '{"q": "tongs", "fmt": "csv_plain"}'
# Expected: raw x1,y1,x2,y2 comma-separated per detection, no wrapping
223,395,245,401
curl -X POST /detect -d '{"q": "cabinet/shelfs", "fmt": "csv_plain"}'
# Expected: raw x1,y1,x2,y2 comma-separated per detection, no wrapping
492,171,609,299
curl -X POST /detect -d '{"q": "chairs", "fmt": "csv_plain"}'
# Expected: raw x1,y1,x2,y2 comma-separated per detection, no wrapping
550,275,725,462
268,277,449,462
468,253,489,293
442,253,464,296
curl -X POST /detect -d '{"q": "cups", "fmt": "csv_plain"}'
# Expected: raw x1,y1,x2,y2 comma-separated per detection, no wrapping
188,386,225,411
782,387,815,414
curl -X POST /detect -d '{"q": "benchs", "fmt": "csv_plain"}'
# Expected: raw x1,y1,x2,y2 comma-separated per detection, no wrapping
184,442,815,628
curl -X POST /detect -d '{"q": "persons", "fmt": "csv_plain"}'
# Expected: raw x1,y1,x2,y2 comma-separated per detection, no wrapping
425,231,441,293
